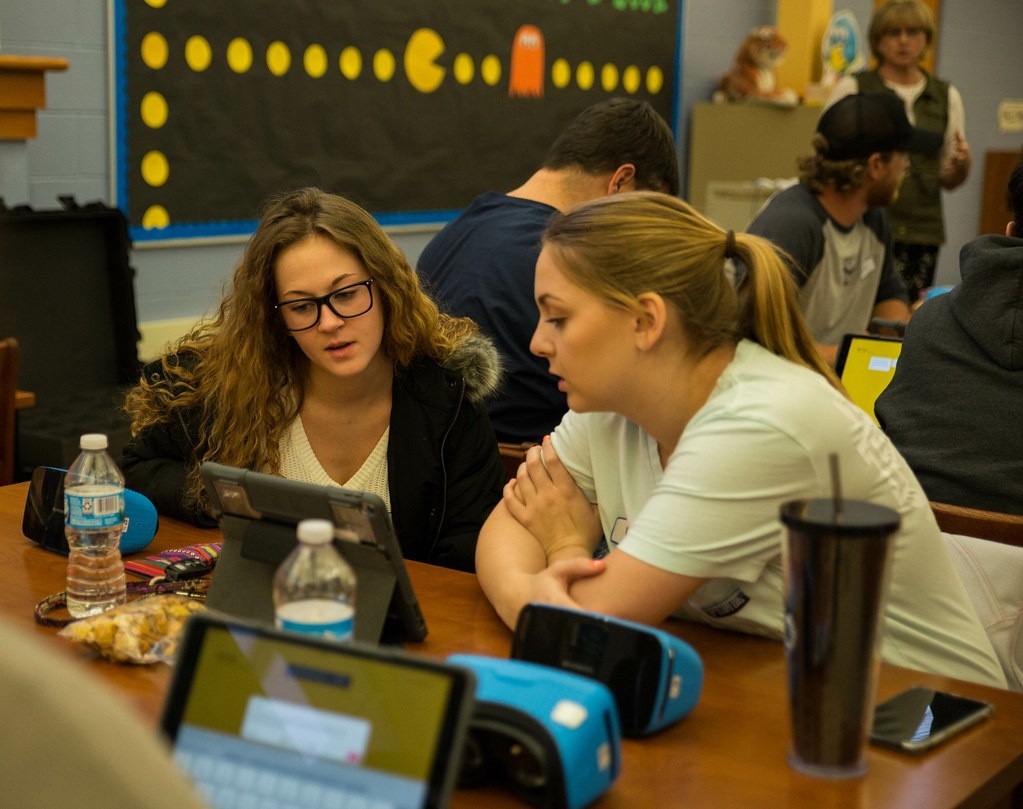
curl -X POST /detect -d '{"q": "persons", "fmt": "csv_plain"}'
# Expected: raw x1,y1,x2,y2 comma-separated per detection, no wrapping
874,159,1023,514
117,188,507,574
475,190,1008,692
413,96,681,574
723,91,943,374
823,0,971,304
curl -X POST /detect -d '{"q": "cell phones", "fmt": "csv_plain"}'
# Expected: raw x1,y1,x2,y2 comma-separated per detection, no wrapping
868,687,993,756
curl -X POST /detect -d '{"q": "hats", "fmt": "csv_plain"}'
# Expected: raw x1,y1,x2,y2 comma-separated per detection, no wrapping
814,92,945,160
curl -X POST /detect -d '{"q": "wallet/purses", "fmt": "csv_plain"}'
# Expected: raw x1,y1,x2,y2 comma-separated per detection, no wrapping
122,537,224,582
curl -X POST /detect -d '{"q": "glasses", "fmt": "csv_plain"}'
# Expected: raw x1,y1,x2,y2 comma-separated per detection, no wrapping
267,276,379,332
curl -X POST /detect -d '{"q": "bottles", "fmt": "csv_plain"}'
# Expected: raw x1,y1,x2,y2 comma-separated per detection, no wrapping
64,434,127,618
272,518,355,645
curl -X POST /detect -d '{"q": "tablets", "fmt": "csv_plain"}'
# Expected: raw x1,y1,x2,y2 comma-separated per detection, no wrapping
161,615,476,809
200,462,429,644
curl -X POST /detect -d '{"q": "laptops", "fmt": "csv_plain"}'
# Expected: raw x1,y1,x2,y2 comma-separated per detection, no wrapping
835,333,904,432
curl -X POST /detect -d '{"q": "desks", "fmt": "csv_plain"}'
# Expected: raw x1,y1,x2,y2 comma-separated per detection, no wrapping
0,475,1022,809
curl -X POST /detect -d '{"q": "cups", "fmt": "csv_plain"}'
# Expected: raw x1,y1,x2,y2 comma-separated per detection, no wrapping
779,497,901,779
